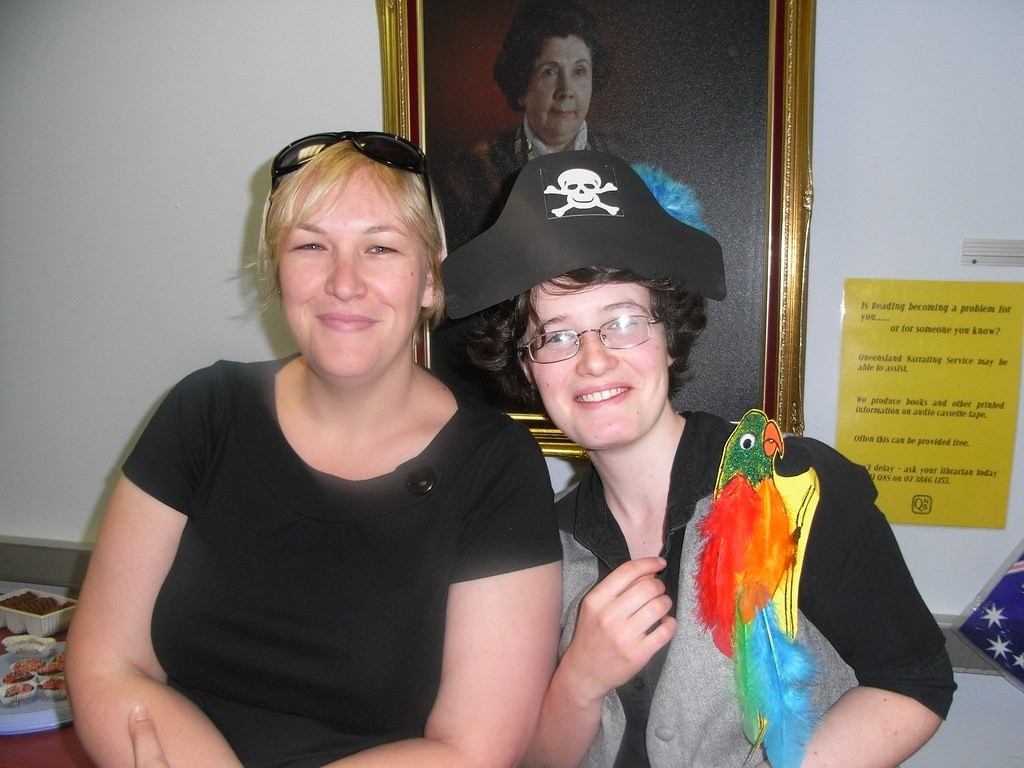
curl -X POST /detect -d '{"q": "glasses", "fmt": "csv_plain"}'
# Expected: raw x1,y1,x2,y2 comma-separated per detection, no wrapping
270,131,434,217
517,315,665,364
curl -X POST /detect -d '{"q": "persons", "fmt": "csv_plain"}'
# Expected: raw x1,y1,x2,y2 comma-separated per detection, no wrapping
64,134,562,767
430,156,956,767
438,6,642,244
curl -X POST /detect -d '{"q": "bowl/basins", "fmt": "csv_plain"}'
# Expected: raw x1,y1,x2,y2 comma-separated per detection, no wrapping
0,588,77,637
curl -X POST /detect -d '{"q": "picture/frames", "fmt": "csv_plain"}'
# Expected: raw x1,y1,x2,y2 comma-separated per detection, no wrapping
375,0,817,459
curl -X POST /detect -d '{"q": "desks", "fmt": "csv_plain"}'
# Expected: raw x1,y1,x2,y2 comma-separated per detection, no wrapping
0,625,97,768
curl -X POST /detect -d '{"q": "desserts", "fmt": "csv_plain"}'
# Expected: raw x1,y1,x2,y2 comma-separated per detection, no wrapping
0,653,68,706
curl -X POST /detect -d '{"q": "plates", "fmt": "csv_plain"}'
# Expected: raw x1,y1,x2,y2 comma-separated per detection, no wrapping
0,639,72,736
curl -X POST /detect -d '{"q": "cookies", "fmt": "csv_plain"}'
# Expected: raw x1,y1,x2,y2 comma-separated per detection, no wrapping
0,591,75,616
